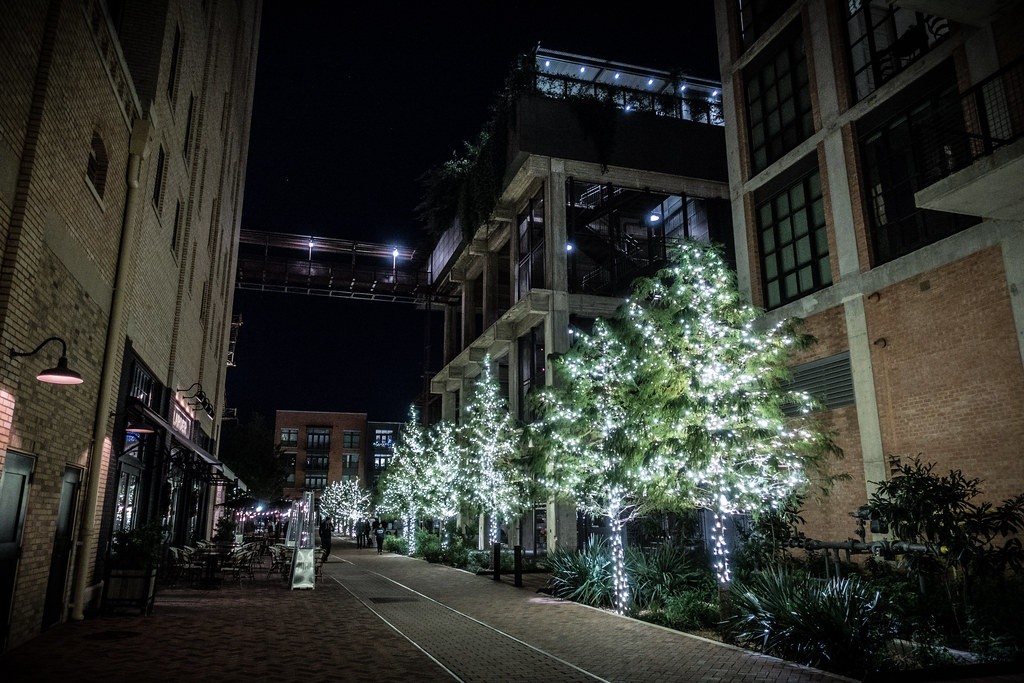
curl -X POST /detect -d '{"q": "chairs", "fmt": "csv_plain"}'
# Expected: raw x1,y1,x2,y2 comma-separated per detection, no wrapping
168,538,327,589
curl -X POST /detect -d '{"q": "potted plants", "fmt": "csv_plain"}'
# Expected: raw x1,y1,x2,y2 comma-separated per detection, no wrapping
98,515,173,617
210,512,240,553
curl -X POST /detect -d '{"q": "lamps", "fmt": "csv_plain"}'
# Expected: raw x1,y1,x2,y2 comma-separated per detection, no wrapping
177,382,216,421
9,335,85,385
110,401,155,434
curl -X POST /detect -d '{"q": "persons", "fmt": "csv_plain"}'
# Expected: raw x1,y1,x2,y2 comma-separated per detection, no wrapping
318,518,336,562
243,516,385,554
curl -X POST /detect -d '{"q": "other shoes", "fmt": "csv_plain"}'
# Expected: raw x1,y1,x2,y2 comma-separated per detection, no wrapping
378,552,382,555
323,557,327,562
